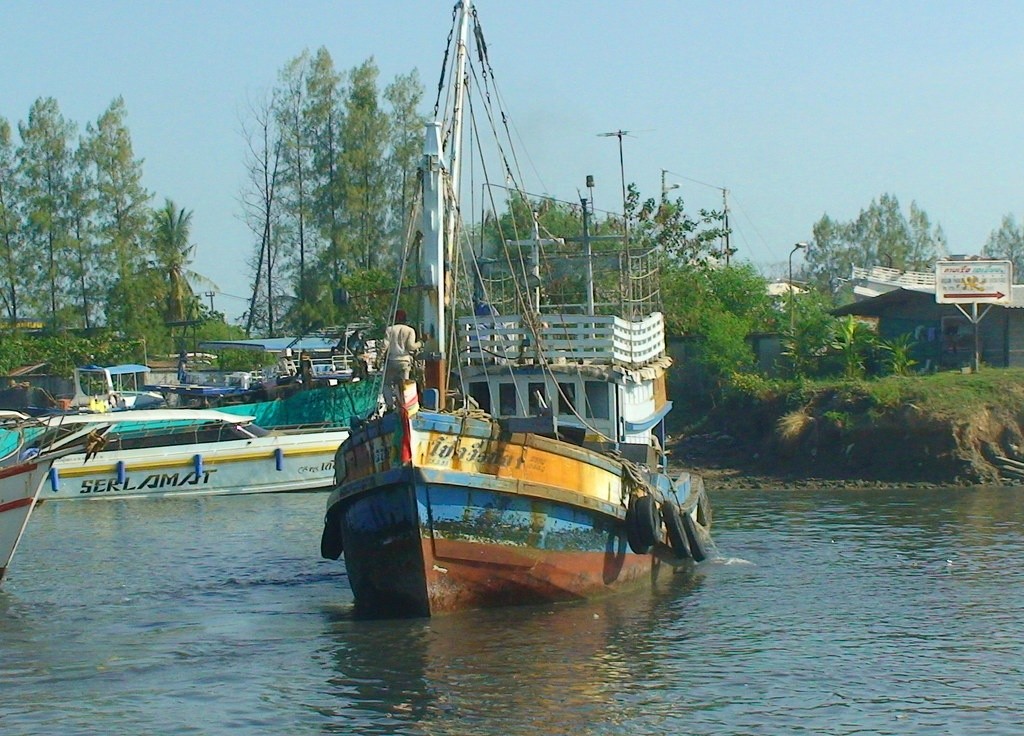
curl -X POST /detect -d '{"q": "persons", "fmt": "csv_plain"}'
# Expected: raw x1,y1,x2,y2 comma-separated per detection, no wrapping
375,309,429,413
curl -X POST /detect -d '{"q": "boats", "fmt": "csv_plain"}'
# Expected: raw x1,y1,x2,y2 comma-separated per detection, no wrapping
0,356,355,515
318,0,711,615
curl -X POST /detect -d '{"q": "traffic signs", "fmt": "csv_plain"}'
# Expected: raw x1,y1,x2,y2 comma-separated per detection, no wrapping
935,258,1014,309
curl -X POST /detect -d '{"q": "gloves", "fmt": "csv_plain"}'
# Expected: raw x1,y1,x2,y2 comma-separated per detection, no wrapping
375,355,384,370
419,340,424,347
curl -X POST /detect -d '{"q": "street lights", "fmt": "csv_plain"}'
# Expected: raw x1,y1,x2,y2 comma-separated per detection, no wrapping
787,242,806,310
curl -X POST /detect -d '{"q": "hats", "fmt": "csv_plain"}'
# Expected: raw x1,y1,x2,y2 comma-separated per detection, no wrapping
391,310,406,320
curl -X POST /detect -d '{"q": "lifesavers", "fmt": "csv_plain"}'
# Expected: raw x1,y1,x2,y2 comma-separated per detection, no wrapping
682,515,707,563
662,500,692,560
627,495,660,557
